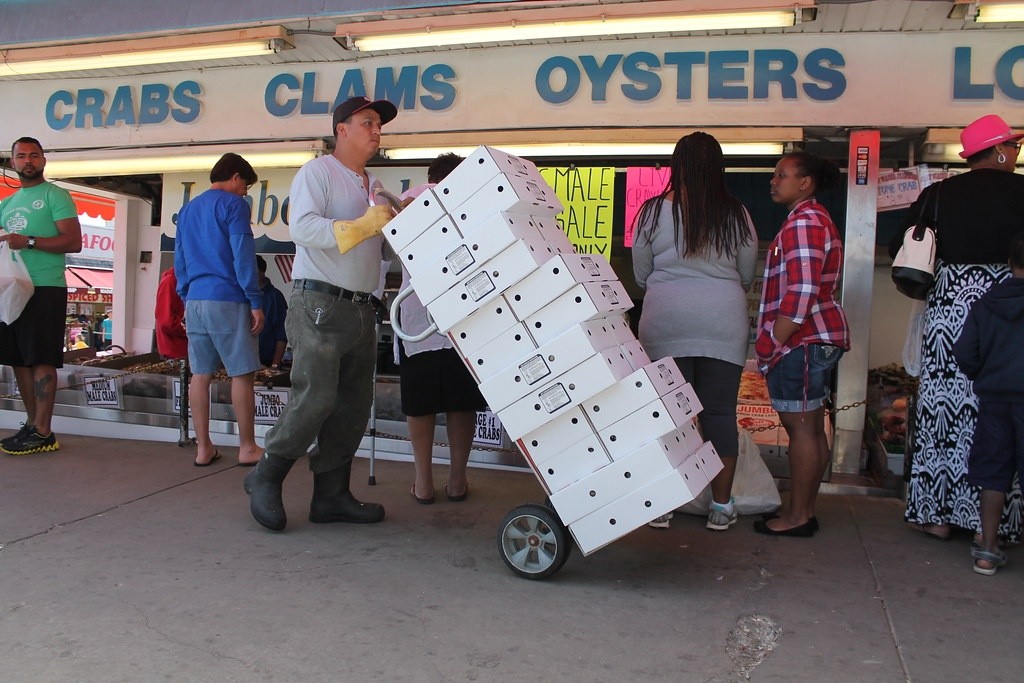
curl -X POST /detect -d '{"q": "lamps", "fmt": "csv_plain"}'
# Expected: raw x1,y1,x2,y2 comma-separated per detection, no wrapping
0,24,295,76
334,0,820,52
915,128,1024,164
375,127,805,160
39,139,337,179
948,0,1024,23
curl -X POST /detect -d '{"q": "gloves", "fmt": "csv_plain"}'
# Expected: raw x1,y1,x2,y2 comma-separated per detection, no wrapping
334,205,392,254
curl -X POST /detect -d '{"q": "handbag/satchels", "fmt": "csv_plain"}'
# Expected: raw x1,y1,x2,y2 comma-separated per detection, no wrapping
681,428,782,516
0,240,35,325
891,179,935,300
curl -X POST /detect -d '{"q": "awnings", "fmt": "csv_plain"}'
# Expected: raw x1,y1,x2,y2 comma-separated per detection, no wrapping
69,268,113,294
64,269,89,293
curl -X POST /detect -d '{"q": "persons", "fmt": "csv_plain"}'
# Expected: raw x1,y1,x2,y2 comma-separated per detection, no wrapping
242,96,414,532
154,268,185,358
956,231,1024,577
0,137,82,454
631,131,758,531
394,153,487,505
753,153,851,537
889,115,1024,549
257,255,288,370
102,310,112,350
174,153,265,466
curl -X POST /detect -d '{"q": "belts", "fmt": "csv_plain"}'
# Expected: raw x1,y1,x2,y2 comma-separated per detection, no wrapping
291,279,381,308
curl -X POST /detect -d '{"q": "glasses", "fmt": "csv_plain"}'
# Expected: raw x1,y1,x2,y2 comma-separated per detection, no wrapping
1001,140,1021,154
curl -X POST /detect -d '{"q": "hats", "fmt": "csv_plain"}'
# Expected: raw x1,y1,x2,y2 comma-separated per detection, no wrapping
255,255,268,272
333,95,397,131
958,115,1024,160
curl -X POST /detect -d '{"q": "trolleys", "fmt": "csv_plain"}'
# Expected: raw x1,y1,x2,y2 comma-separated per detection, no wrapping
367,185,628,580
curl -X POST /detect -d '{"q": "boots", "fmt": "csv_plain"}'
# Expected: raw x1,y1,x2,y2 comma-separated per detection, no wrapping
308,459,385,523
242,450,298,531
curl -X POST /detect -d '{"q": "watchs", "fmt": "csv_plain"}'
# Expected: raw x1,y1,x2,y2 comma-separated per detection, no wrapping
27,236,35,249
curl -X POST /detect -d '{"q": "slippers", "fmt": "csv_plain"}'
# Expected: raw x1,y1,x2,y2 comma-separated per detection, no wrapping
444,481,467,501
908,523,951,541
409,480,435,503
239,449,268,467
194,448,220,467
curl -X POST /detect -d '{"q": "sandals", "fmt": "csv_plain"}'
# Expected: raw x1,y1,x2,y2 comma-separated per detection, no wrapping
973,548,1008,576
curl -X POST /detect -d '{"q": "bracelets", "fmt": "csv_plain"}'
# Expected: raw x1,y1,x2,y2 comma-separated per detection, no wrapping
272,364,279,367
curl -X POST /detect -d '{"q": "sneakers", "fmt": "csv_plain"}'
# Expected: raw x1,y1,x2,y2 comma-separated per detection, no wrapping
648,512,673,528
0,421,32,445
0,425,59,455
707,508,737,530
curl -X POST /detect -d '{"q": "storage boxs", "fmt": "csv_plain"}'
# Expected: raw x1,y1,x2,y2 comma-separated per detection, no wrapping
380,145,832,557
864,417,905,474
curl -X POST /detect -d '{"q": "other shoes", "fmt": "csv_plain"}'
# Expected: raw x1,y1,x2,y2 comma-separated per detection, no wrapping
753,514,819,538
